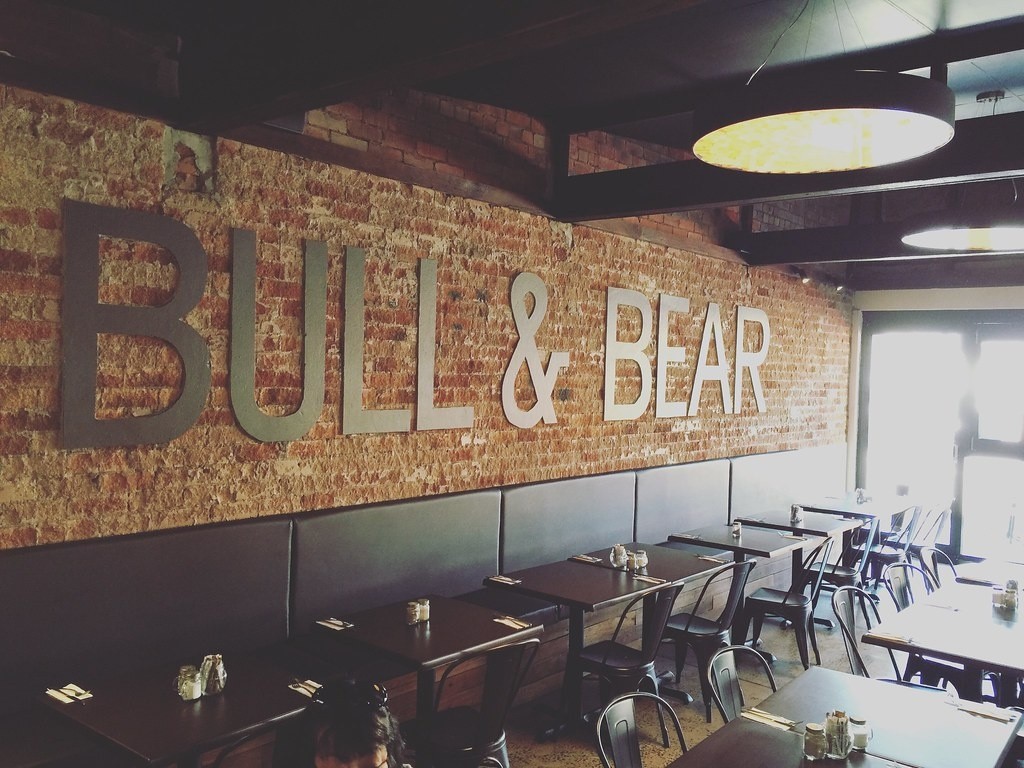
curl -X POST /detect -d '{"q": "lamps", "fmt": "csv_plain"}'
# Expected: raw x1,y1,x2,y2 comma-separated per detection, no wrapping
900,177,1024,253
692,0,957,177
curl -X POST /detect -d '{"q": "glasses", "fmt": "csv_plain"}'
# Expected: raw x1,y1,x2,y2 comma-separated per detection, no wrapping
302,678,389,714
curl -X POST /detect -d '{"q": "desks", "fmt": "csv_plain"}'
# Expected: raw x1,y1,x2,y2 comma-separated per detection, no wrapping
31,498,1024,768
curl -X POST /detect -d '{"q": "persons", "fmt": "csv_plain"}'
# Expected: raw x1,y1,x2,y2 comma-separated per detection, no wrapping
288,688,417,768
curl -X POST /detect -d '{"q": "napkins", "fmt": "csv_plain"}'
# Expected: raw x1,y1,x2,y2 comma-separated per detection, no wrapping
778,533,809,542
572,554,602,563
956,698,1015,723
740,707,796,733
314,617,355,632
488,575,523,585
671,533,702,541
45,682,94,704
865,624,912,643
697,554,726,564
492,615,532,630
631,575,665,584
286,681,325,703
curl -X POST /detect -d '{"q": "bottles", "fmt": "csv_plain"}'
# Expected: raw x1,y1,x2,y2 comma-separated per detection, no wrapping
991,580,1019,610
609,544,648,572
789,505,804,528
732,522,741,539
405,602,421,626
801,708,873,761
177,654,227,701
416,598,430,622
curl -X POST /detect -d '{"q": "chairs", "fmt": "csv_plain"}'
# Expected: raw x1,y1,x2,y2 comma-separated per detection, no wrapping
209,496,968,768
